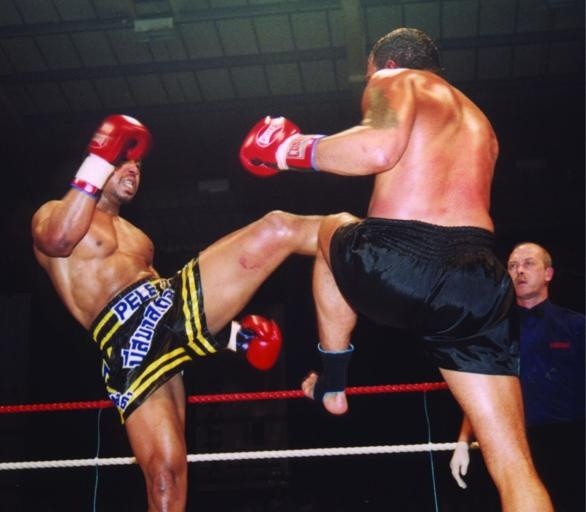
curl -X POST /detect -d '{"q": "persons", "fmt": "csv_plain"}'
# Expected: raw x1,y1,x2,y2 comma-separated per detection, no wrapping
27,112,324,511
237,26,557,512
448,241,585,511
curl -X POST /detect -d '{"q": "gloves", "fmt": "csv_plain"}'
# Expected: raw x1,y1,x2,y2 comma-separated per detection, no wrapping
211,313,284,375
449,440,472,490
67,111,152,201
236,113,330,179
